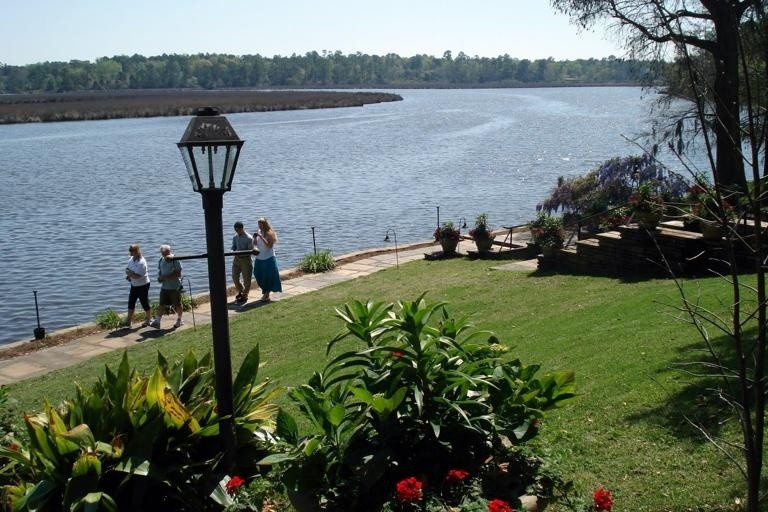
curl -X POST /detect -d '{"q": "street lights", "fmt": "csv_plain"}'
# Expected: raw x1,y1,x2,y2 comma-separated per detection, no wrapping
173,102,247,475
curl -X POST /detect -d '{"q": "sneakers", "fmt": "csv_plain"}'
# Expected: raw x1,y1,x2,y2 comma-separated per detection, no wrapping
119,319,184,329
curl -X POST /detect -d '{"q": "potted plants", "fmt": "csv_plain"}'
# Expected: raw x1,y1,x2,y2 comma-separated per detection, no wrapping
469,213,498,253
529,170,736,256
433,221,462,253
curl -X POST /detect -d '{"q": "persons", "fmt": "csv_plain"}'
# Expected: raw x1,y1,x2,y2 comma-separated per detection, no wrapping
252,217,282,304
226,219,256,306
148,243,184,331
116,243,153,328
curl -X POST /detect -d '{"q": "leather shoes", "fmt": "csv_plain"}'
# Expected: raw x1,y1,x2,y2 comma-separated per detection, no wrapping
236,292,270,303
